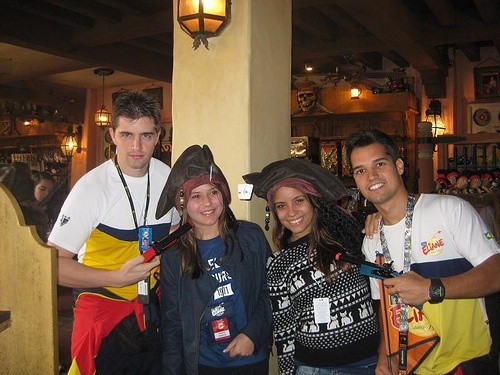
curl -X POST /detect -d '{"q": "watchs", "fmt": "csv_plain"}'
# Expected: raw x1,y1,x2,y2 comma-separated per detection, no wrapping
429,277,445,304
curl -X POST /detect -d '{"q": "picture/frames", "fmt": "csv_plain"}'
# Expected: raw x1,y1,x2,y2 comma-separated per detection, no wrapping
143,87,163,110
474,66,500,102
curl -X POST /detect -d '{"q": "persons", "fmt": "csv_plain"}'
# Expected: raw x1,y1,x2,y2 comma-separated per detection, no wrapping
47,90,177,375
157,145,274,375
434,168,500,197
346,129,500,375
0,161,65,238
242,157,382,375
489,76,497,93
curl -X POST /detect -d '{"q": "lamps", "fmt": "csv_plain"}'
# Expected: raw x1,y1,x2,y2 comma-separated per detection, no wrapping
60,124,82,158
94,68,114,130
350,81,362,100
425,98,446,137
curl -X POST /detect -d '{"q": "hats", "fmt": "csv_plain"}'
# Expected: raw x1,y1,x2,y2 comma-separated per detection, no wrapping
156,144,231,220
242,157,354,216
